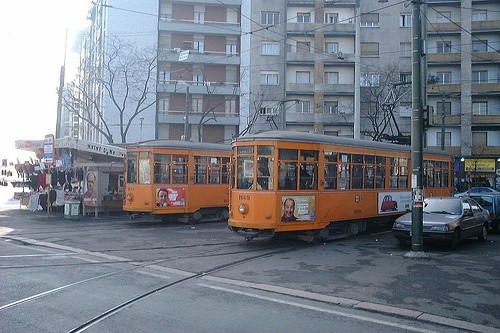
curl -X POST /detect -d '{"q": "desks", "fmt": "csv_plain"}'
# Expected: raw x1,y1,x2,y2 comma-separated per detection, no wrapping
83,204,100,218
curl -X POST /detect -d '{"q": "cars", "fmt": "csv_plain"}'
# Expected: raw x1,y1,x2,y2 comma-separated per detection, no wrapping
392,195,491,252
454,186,500,233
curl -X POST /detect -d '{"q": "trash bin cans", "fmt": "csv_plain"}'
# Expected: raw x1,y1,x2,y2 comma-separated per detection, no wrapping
64,200,82,219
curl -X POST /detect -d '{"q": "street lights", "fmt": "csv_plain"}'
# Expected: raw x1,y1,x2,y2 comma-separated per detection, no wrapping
139,116,144,140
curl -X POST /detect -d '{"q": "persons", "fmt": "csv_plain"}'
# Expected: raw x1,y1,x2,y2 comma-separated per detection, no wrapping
257,167,268,190
84,172,96,198
157,189,170,206
280,199,299,222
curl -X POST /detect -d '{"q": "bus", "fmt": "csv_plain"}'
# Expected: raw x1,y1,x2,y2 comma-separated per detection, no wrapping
122,140,233,225
221,130,455,242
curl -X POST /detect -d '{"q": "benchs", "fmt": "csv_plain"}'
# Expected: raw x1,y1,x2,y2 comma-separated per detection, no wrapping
102,200,123,216
